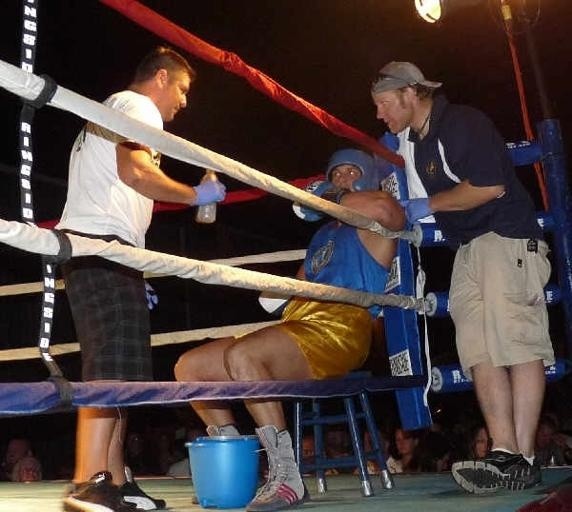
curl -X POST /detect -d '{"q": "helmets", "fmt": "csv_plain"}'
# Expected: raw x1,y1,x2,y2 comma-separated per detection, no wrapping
325,148,381,192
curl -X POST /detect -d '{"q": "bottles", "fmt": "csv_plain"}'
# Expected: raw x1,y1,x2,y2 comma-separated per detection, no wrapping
195,168,218,223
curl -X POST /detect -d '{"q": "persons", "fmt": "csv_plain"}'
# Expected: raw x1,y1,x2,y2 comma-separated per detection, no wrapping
53,43,229,512
175,145,402,512
367,61,555,495
1,411,568,485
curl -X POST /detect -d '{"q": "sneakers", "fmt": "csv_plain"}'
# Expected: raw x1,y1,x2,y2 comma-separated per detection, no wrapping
117,465,166,511
63,470,142,512
529,454,542,487
449,448,535,495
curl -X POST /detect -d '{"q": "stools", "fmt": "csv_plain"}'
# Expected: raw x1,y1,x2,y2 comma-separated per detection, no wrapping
293,375,395,499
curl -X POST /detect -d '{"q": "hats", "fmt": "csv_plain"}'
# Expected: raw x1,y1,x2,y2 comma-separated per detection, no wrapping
370,59,444,93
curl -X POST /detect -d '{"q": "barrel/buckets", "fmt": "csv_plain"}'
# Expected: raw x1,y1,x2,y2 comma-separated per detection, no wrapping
184,433,261,507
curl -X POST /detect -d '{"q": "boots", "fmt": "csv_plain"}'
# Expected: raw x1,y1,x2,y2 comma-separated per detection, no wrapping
246,425,311,511
192,424,267,505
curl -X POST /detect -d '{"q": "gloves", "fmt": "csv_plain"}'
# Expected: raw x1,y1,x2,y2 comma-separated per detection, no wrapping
399,198,433,226
292,178,348,223
145,282,159,310
258,291,293,314
195,180,228,208
